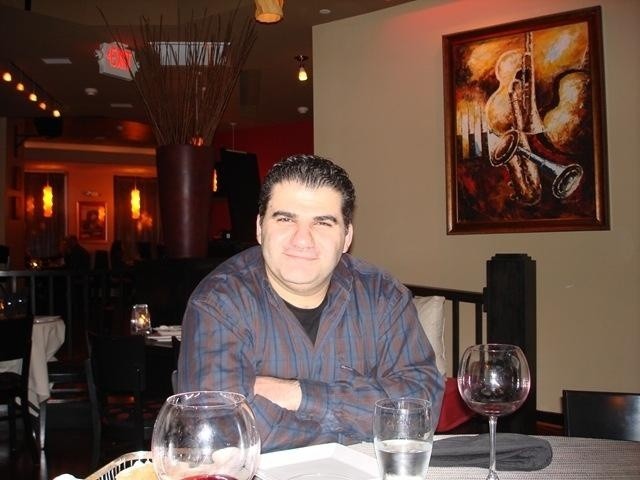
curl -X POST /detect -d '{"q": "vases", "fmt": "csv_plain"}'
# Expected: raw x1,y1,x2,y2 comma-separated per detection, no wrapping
154,136,215,264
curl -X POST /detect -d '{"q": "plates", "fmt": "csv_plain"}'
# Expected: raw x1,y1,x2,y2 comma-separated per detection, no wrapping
253,441,384,480
147,326,181,345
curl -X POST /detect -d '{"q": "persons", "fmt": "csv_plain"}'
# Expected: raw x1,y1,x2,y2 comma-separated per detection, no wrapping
48,237,69,268
51,235,90,276
174,153,448,454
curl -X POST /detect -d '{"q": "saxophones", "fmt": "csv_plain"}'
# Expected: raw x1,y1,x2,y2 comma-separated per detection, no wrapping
489,31,541,205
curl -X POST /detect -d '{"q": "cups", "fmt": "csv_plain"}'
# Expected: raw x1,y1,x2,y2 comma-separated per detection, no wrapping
152,391,261,480
373,398,433,477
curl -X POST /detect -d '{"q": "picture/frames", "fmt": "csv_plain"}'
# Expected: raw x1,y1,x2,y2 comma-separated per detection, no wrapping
77,199,111,245
437,2,615,237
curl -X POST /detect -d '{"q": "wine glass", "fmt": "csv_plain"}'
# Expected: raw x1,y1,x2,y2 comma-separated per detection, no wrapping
458,344,532,479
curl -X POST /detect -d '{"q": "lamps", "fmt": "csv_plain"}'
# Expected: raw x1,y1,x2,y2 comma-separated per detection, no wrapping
131,179,143,223
1,55,62,118
42,170,54,222
292,51,311,84
253,0,288,27
